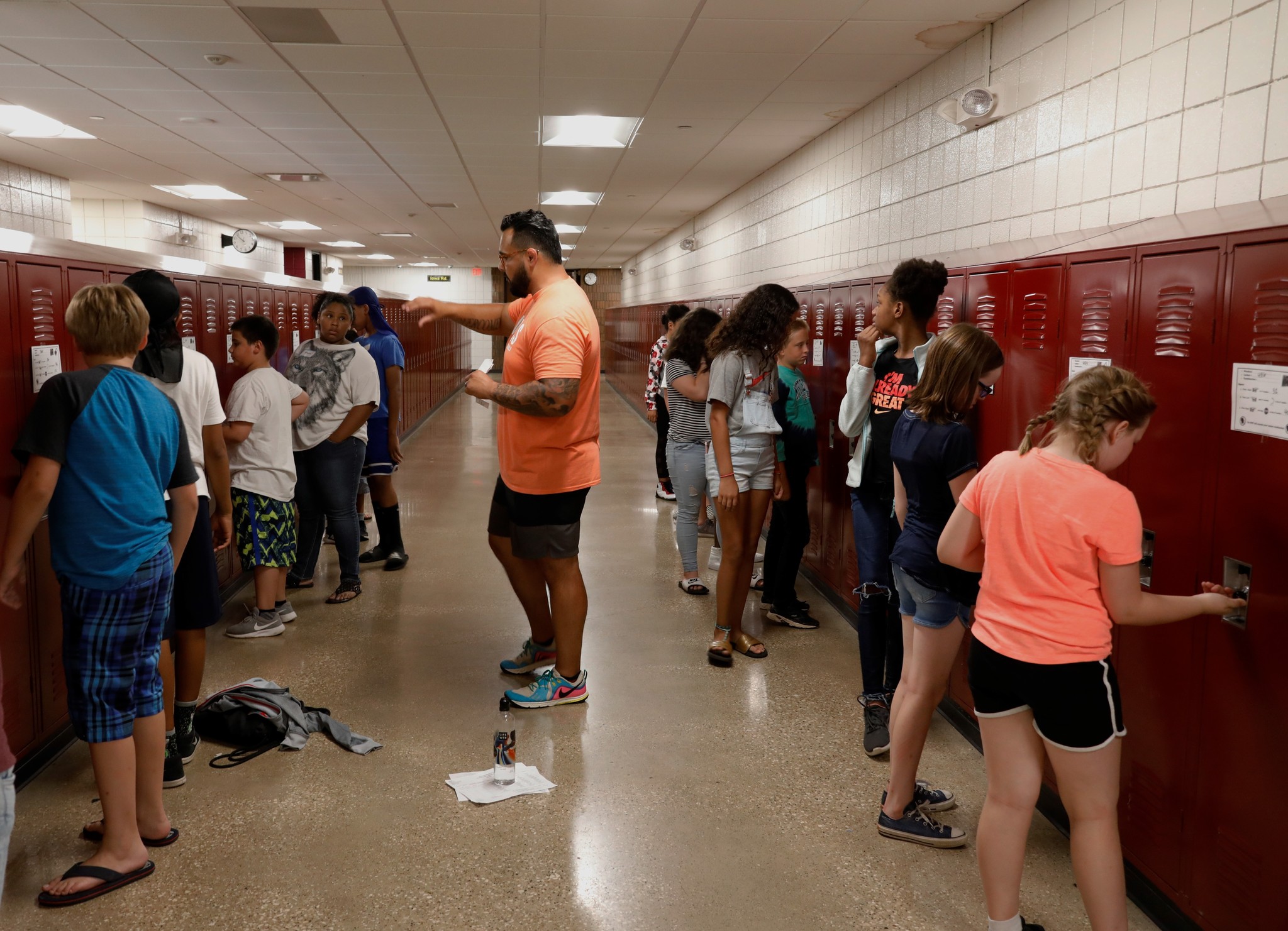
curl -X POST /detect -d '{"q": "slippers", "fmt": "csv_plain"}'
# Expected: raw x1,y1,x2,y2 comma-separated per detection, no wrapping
708,640,733,661
83,818,180,847
359,545,388,563
678,577,709,594
730,632,768,658
749,573,764,589
37,859,156,905
382,551,409,572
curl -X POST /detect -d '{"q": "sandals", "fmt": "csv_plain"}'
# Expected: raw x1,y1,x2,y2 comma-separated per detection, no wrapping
328,581,362,603
286,572,314,589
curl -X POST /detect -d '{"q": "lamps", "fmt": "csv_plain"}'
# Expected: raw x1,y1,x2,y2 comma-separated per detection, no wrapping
680,238,697,251
175,232,198,246
627,268,637,276
325,267,335,275
936,82,1005,131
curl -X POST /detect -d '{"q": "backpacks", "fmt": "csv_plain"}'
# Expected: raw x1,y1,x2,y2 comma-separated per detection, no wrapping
192,700,331,768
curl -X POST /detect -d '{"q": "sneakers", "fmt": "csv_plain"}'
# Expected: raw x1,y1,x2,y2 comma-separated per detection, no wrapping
161,722,201,788
358,519,370,542
673,515,715,538
500,636,558,675
323,524,336,544
708,546,764,571
857,685,899,756
655,479,676,501
877,794,969,848
224,600,298,638
880,780,956,812
504,664,589,708
759,589,820,629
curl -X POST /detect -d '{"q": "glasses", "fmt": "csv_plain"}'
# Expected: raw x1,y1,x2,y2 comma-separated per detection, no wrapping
498,248,539,266
978,380,993,398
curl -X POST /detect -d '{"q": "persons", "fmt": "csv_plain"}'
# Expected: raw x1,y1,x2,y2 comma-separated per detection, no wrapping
222,315,310,638
283,285,409,604
838,258,948,756
119,269,232,789
641,305,821,628
0,283,199,909
706,284,800,662
0,647,16,901
877,325,1006,849
937,366,1249,930
402,209,601,708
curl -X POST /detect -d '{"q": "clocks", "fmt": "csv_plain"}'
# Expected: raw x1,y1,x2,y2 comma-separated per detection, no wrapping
584,272,597,285
221,229,258,254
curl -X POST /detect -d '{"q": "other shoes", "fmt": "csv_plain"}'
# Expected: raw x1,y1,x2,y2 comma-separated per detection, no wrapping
1020,915,1045,931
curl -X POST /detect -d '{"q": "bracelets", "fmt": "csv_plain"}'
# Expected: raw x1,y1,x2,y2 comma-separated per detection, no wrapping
700,361,706,364
720,472,735,477
774,470,781,474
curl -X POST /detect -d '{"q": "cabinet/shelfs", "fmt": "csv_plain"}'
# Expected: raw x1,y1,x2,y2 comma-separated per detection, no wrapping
0,228,472,793
604,193,1286,930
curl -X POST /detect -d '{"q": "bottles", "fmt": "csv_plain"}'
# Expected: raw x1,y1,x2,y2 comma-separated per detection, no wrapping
493,697,516,786
